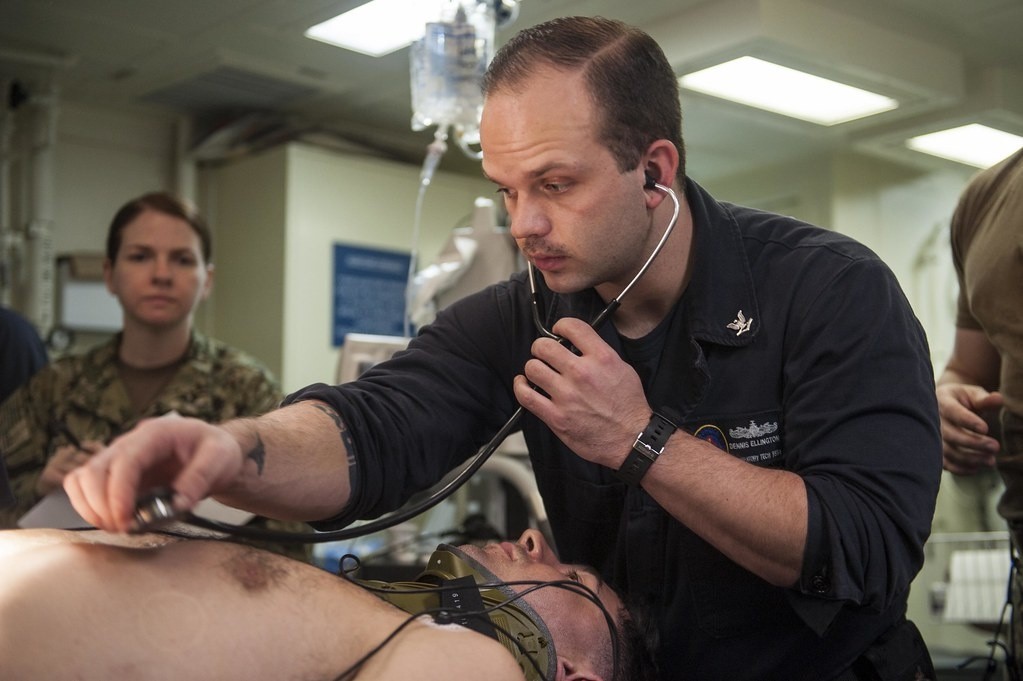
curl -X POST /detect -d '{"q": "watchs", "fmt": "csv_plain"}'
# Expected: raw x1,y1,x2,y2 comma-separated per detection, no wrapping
612,410,680,486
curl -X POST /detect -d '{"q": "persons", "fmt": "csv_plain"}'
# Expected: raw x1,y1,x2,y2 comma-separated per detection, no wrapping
0,191,289,507
59,13,945,681
0,265,50,405
0,525,658,681
936,146,1023,681
